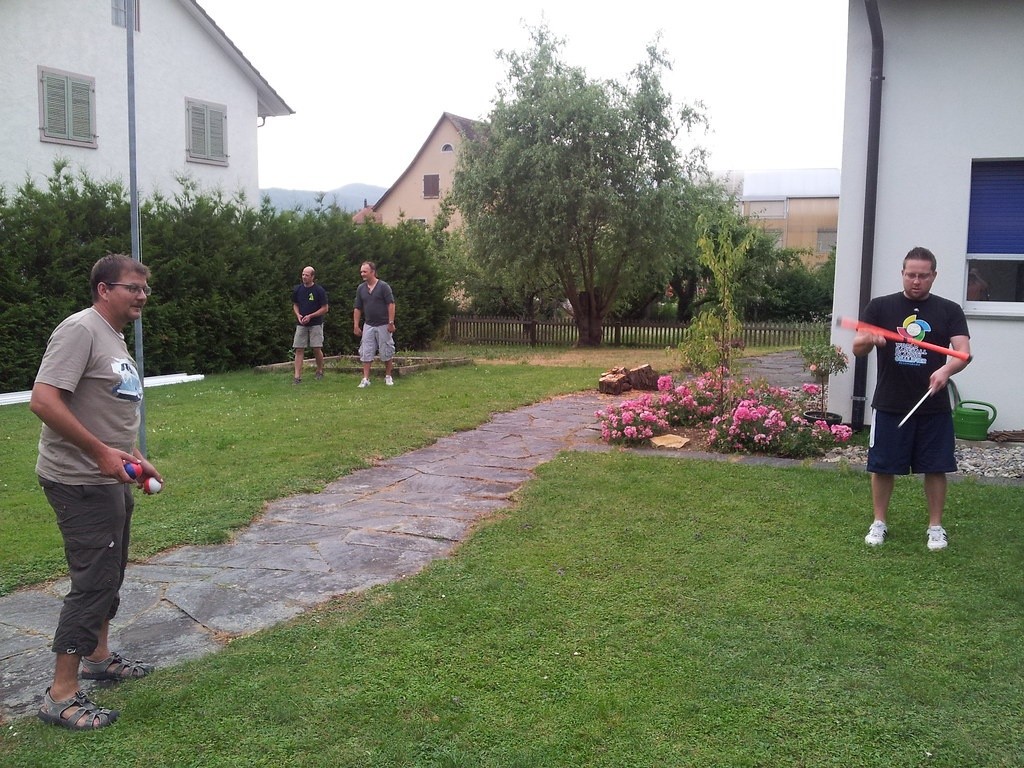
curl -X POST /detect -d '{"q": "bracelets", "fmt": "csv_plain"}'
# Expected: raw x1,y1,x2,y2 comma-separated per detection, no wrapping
389,321,394,324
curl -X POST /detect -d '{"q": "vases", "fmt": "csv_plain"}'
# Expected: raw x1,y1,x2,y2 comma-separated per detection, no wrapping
803,411,842,428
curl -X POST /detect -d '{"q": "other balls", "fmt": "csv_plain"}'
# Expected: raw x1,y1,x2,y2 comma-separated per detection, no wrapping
125,462,143,479
143,476,162,494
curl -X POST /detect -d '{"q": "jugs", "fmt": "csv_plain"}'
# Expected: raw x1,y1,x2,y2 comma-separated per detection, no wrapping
951,399,997,441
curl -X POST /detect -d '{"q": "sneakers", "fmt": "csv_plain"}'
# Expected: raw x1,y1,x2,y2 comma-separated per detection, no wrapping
927,526,949,549
385,375,394,385
864,520,887,546
357,378,371,388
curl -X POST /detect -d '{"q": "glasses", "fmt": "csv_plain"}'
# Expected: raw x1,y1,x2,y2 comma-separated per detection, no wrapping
108,282,151,296
902,270,933,280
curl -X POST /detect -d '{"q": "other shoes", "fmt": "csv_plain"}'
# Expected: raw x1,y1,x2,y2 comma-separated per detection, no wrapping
294,377,302,383
316,371,323,380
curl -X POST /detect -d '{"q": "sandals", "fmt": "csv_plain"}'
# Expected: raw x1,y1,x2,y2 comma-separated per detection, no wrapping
37,686,119,729
81,651,154,680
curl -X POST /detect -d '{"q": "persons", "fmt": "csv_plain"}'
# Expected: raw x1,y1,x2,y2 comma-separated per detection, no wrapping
353,261,395,388
28,255,165,730
852,246,973,551
290,265,328,386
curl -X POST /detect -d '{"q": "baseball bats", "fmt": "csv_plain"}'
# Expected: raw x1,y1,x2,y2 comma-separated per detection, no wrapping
836,316,973,363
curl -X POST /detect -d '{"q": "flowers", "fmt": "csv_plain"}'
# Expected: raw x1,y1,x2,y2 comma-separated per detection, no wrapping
592,336,851,458
